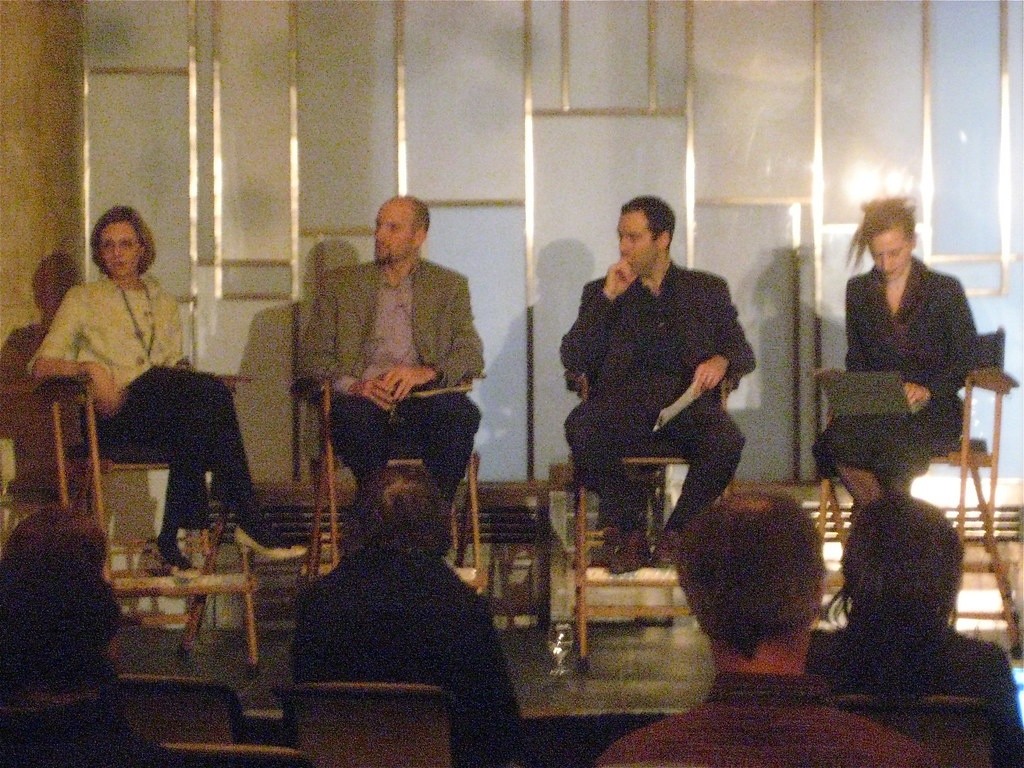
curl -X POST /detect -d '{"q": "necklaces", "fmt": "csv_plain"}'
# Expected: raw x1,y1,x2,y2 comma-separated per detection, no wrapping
117,278,156,357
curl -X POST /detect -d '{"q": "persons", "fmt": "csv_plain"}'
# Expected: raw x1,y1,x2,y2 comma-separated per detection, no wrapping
811,197,980,509
0,505,194,768
298,197,481,507
560,195,757,572
289,462,530,768
27,204,308,580
591,476,1024,768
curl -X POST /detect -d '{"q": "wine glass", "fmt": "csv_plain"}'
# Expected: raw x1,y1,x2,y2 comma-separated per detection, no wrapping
548,622,572,674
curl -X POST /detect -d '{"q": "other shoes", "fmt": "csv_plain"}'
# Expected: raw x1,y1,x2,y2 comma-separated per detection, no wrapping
610,529,678,572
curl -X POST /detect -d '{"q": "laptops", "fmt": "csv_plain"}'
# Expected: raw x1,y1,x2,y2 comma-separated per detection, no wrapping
819,369,926,415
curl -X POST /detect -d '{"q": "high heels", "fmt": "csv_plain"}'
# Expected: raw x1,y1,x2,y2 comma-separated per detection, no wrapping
235,527,308,560
151,540,199,580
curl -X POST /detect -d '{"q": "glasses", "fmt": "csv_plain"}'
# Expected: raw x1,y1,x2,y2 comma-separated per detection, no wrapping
99,239,138,252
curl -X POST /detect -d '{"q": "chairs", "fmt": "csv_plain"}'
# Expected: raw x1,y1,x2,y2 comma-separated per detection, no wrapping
303,380,488,595
547,371,728,665
115,673,254,747
33,372,261,672
806,366,1024,662
826,693,993,768
278,681,455,768
